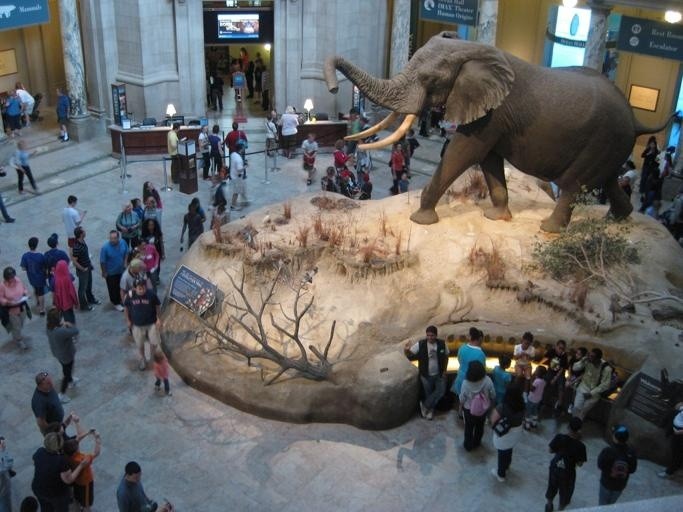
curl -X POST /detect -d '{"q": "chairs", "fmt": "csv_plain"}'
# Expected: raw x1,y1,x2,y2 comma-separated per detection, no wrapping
187,119,201,126
316,112,329,121
142,117,157,127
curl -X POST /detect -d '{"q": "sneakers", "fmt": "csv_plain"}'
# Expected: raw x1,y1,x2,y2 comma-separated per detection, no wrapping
18,190,43,196
67,373,83,389
419,401,434,420
58,393,72,403
267,152,292,159
89,298,102,305
491,464,511,483
58,135,69,143
207,94,269,111
35,297,46,316
656,467,677,479
15,339,28,351
5,218,15,223
307,179,311,185
554,400,574,414
544,503,553,511
81,305,97,312
202,173,248,182
164,390,172,396
7,119,35,139
112,303,125,312
138,358,147,371
231,200,252,211
154,388,162,393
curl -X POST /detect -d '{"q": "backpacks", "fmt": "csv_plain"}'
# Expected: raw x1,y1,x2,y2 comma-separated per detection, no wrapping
598,362,617,397
303,152,315,166
389,159,393,168
549,454,575,478
607,457,630,481
469,392,492,416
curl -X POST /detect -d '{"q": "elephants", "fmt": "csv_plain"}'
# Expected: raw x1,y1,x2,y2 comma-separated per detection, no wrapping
323,30,681,234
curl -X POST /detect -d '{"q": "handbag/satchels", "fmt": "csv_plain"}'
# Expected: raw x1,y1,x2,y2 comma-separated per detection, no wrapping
124,290,133,304
275,134,279,143
116,221,123,232
235,139,248,149
492,415,511,437
68,499,83,511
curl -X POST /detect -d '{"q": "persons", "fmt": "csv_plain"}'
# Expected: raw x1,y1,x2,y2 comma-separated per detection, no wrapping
545,418,638,512
596,131,682,249
5,82,41,195
0,182,173,512
204,47,274,105
405,326,614,480
56,87,70,140
243,23,255,35
660,382,682,477
168,105,448,249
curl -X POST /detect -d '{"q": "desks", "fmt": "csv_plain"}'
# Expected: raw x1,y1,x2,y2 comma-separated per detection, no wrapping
108,122,208,163
290,119,348,156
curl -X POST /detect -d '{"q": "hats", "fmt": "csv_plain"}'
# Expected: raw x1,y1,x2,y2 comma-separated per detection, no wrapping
569,415,583,432
611,423,629,441
47,232,59,247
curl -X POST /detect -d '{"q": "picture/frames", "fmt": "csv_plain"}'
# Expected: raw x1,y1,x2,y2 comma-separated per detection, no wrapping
0,46,19,79
628,83,661,114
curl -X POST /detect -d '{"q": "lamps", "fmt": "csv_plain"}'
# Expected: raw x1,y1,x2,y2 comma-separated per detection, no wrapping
164,103,176,129
302,98,313,124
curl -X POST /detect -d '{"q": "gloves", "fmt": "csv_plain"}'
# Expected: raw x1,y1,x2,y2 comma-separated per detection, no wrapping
582,393,592,400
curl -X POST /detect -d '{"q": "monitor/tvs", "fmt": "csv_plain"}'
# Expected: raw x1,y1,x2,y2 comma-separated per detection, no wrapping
216,12,261,42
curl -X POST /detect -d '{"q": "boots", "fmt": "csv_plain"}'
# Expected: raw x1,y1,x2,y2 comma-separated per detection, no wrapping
522,415,539,435
